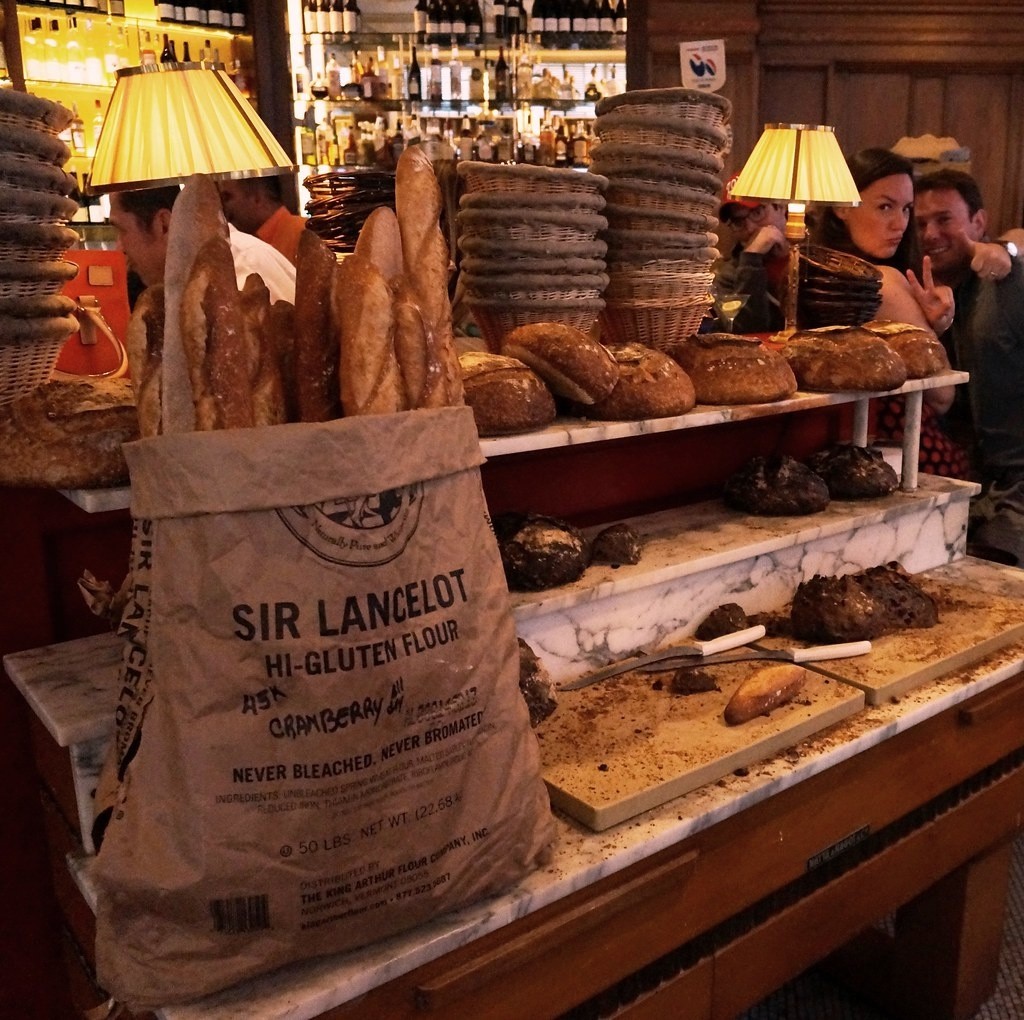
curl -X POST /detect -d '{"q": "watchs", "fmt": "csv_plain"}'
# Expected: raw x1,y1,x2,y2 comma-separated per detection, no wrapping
994,240,1018,264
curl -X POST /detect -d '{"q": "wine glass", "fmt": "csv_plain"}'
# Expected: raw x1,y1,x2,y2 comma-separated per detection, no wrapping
716,295,751,334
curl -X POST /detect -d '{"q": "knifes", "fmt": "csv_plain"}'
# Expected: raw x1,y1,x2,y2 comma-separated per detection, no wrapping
557,625,767,690
635,640,871,671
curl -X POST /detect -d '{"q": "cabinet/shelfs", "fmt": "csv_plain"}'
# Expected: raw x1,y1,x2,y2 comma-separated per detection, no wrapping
0,0,628,250
0,373,1024,1020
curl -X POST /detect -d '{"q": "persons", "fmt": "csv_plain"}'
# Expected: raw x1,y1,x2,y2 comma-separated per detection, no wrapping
714,145,1024,569
220,176,309,269
108,183,297,310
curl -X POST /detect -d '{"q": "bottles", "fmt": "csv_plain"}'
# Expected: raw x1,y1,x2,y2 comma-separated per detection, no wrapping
0,0,257,156
300,0,627,170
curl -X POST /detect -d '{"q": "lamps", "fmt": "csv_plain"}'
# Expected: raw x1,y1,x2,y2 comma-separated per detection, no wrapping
727,123,863,338
85,62,301,196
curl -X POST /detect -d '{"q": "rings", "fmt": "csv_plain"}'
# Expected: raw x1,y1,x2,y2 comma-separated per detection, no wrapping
989,271,998,278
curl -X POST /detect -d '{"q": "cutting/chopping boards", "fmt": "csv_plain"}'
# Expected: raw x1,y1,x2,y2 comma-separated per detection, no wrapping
750,574,1024,706
534,635,865,830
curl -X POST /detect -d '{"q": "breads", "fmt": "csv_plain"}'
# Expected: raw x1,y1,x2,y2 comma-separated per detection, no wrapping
673,665,718,694
0,377,140,491
725,443,898,516
450,322,948,437
697,566,939,647
490,510,643,591
128,144,466,442
724,664,807,725
516,637,557,728
